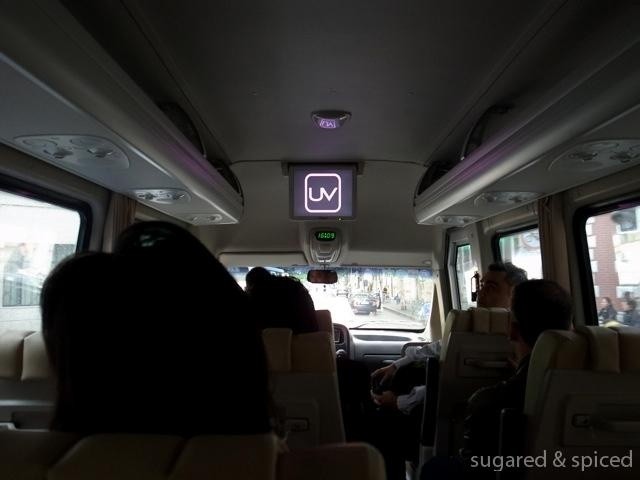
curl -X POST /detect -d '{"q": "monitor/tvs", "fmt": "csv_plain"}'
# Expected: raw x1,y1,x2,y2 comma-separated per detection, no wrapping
288,162,357,221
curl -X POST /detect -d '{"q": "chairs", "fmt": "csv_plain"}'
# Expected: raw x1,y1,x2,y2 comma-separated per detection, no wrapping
0,304,640,479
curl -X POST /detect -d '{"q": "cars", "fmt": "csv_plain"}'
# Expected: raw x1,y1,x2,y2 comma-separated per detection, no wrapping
348,292,381,314
336,286,348,297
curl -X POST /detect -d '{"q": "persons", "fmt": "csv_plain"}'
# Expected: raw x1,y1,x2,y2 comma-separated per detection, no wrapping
370,262,528,415
309,270,333,283
376,286,431,326
418,279,574,480
597,291,640,329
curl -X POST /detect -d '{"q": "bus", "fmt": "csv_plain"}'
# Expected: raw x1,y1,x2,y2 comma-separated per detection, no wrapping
225,265,290,294
